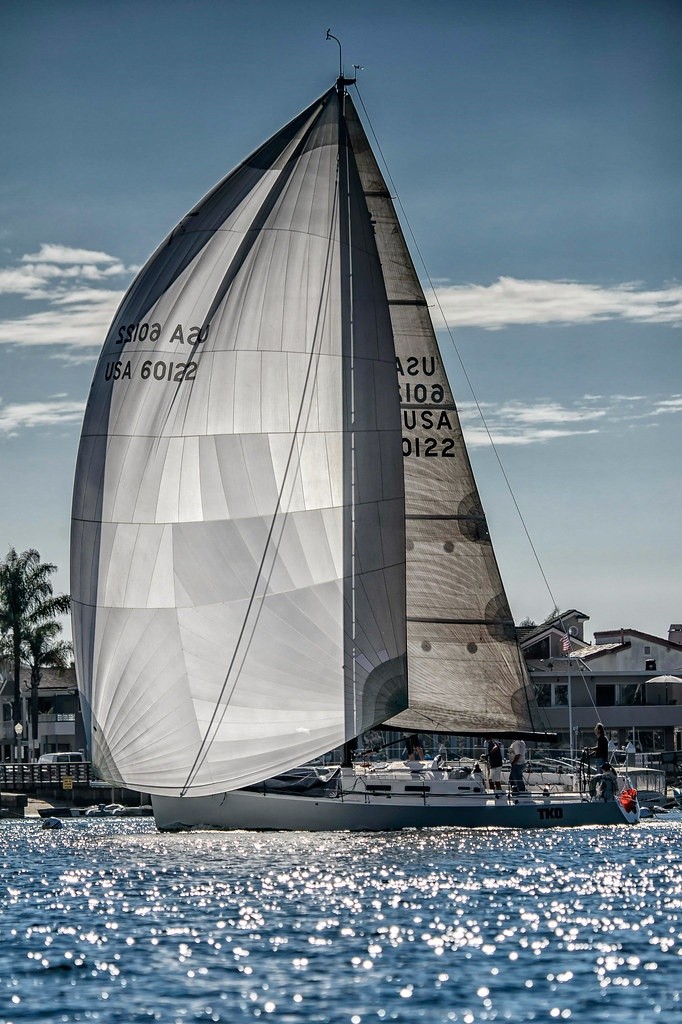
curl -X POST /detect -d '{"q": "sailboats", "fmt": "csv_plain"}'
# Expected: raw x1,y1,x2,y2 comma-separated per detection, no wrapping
71,29,640,834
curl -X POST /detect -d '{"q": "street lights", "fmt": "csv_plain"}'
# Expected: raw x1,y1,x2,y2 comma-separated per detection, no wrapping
14,722,23,773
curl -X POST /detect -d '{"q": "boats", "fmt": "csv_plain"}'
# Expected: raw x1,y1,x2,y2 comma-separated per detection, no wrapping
37,807,70,818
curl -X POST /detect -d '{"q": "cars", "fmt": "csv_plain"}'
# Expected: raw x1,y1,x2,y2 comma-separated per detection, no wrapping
37,752,86,779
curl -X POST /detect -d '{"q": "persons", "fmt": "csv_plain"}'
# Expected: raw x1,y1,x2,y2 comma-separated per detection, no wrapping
403,733,424,760
596,762,618,798
622,736,636,766
439,741,449,753
590,723,609,774
479,738,502,790
508,740,526,791
